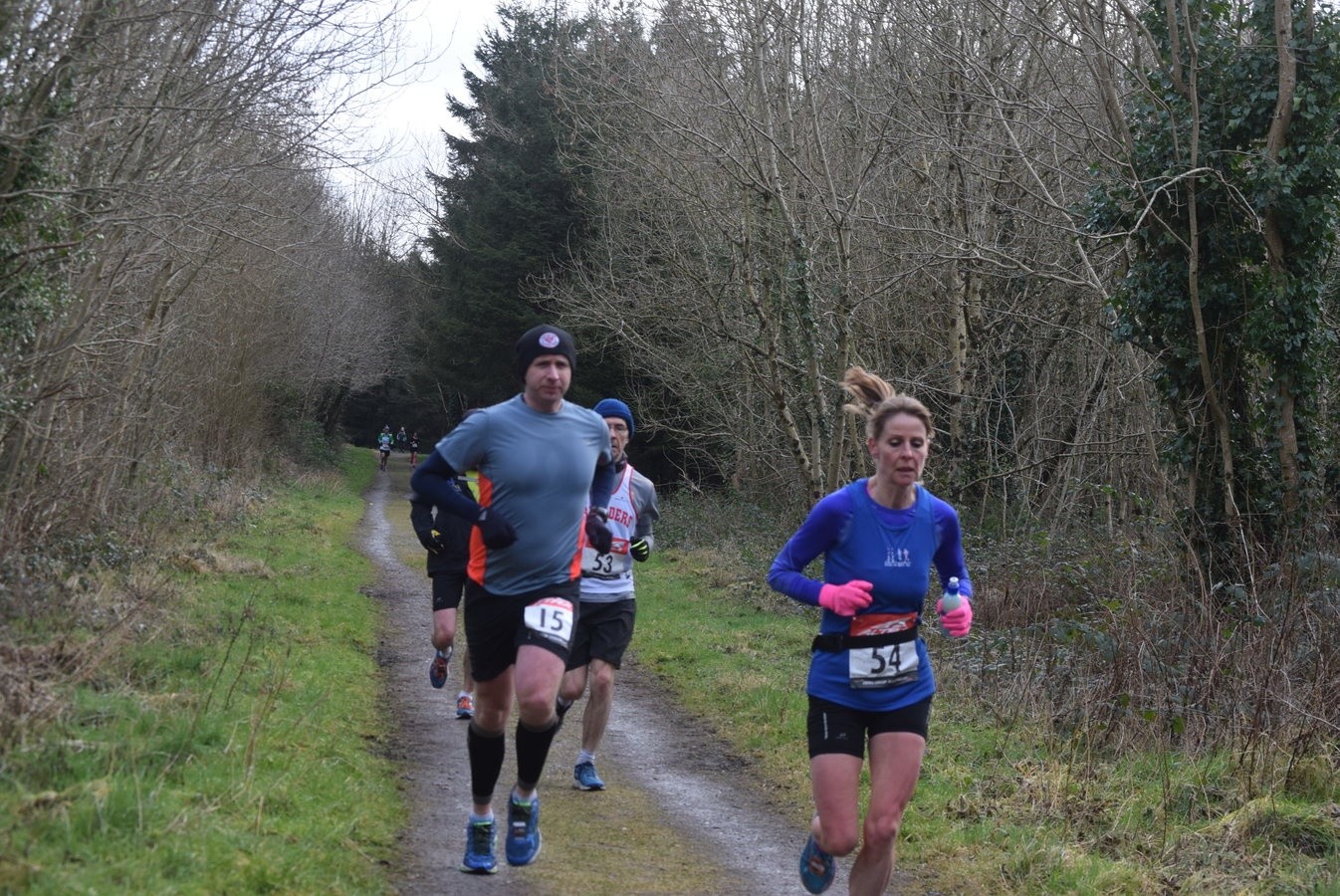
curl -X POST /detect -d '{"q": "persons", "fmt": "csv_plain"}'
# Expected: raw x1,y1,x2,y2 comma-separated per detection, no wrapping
409,471,482,719
377,426,420,473
554,399,661,791
410,323,616,875
765,364,973,896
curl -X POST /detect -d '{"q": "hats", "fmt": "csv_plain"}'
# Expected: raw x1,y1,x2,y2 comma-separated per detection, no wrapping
594,399,634,439
516,325,576,377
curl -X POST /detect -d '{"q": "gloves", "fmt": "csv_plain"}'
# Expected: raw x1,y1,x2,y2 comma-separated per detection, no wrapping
585,516,612,554
630,536,649,562
937,595,973,636
480,511,517,550
819,580,873,615
418,529,444,556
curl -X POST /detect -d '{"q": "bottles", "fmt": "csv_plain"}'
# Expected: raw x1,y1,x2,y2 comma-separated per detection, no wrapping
939,577,962,637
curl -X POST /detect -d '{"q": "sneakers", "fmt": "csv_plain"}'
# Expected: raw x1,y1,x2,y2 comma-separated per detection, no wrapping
553,697,573,733
429,643,454,688
573,761,604,791
457,696,475,718
799,838,835,894
505,784,541,865
460,815,498,873
